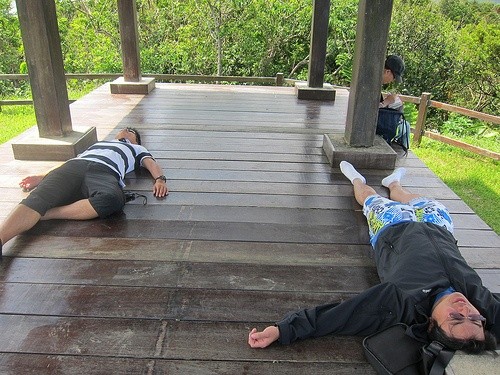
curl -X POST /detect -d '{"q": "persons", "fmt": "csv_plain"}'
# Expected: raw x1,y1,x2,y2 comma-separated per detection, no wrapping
0,126,169,263
376,55,410,158
247,161,500,356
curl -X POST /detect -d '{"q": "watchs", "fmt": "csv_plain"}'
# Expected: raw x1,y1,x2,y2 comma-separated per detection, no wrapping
155,174,166,183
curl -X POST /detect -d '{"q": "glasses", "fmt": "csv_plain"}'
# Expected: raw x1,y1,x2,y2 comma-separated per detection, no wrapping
439,312,486,329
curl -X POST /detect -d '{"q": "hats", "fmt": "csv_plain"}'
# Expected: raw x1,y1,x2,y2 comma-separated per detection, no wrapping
387,55,405,83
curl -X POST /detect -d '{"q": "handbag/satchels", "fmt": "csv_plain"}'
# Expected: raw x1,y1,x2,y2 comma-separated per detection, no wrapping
376,107,403,143
362,323,456,375
